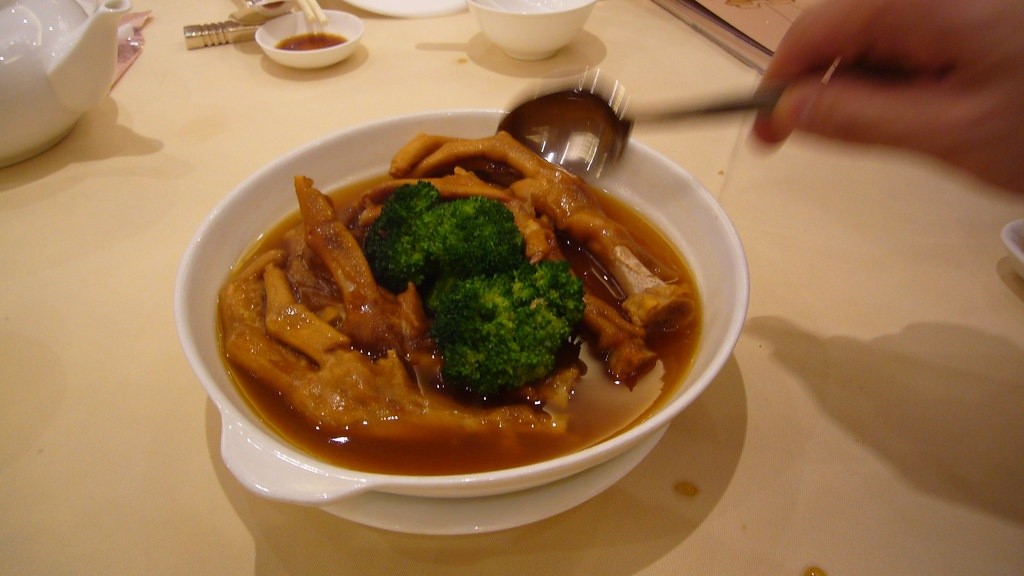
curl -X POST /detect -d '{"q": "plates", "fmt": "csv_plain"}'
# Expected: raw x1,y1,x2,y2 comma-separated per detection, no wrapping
319,426,677,537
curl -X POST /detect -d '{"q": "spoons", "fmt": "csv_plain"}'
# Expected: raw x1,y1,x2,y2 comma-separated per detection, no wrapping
228,0,294,26
494,59,786,178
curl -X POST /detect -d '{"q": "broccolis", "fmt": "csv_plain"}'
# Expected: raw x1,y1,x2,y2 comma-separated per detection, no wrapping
364,179,587,402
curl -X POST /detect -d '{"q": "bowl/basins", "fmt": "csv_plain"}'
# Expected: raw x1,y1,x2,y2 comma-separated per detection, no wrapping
170,109,751,508
254,9,364,73
469,0,596,62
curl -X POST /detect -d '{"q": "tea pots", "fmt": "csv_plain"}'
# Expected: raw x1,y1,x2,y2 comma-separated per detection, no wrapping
0,0,135,168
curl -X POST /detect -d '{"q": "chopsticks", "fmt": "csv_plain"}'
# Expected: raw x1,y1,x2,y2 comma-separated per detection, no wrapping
294,0,328,24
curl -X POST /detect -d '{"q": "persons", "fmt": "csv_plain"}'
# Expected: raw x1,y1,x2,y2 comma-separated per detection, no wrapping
750,0,1024,198
